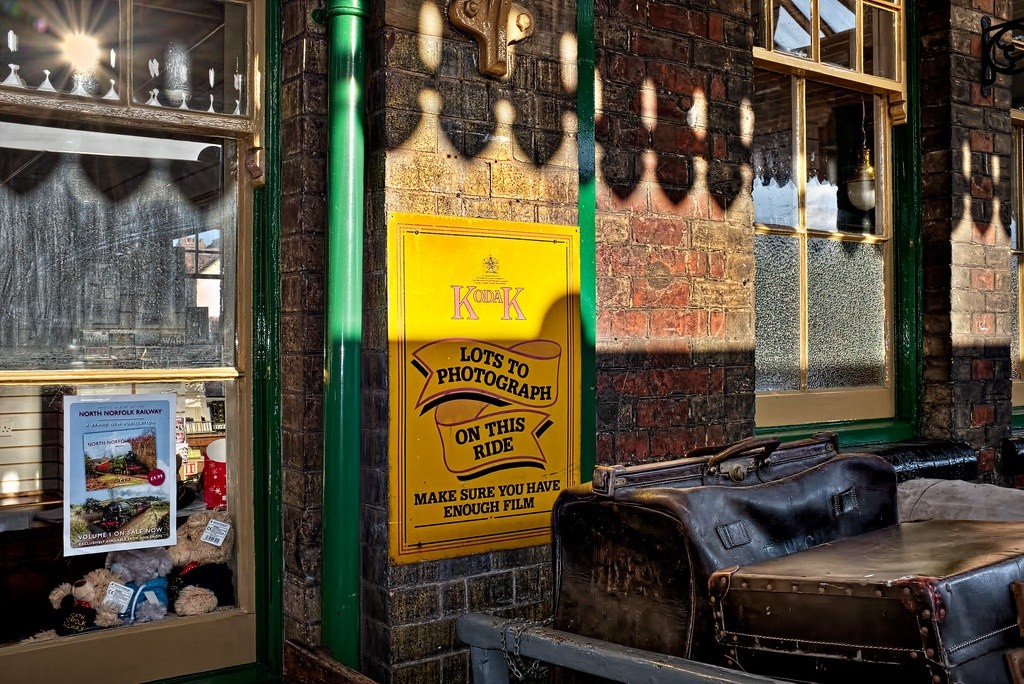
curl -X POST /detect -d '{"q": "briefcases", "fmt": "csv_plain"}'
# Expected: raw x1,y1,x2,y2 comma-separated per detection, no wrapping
707,517,1024,684
550,431,899,659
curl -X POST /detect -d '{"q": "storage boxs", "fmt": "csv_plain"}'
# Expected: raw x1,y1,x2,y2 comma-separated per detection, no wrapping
705,517,1024,683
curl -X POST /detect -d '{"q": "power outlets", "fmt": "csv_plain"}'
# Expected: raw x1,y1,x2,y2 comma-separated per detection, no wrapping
0,420,12,436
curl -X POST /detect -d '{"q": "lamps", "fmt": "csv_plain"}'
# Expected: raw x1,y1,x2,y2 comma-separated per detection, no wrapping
845,94,874,211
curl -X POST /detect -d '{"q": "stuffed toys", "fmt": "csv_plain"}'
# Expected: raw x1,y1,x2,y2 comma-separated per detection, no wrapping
161,508,240,616
102,540,172,626
20,568,126,646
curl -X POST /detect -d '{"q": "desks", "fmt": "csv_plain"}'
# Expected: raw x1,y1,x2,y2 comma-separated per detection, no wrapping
186,432,225,454
